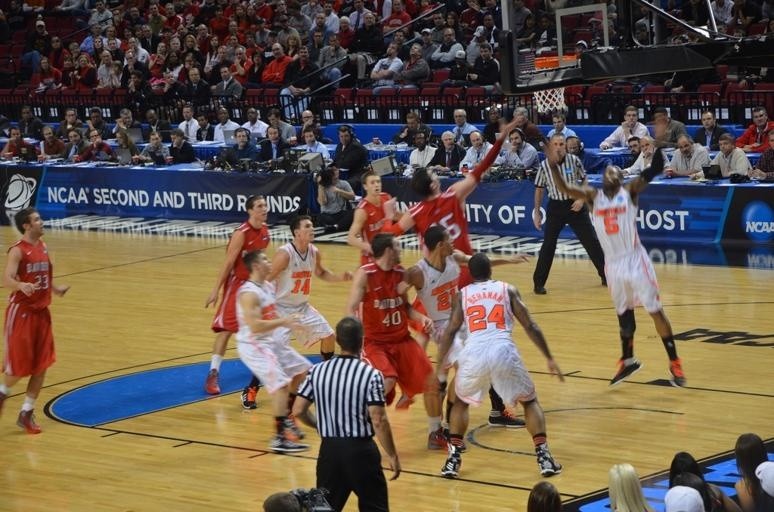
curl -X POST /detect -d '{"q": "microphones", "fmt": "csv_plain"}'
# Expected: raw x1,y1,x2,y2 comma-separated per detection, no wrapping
479,141,484,145
312,139,317,142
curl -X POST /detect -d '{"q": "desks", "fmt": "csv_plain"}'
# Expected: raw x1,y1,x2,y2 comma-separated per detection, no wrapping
1,123,364,227
361,123,774,249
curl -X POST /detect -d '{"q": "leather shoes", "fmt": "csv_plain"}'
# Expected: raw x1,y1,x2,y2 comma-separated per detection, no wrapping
532,285,547,296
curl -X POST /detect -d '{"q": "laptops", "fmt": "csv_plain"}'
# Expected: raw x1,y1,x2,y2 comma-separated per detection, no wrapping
148,150,165,164
702,165,729,179
251,132,261,144
126,128,147,143
223,129,238,144
115,148,139,164
431,134,443,147
457,134,472,147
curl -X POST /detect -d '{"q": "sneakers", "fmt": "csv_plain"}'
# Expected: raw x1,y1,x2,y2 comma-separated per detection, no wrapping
266,435,312,451
426,428,467,452
486,409,527,428
535,451,565,475
203,369,220,395
608,356,644,389
16,407,42,434
665,359,688,390
393,393,415,411
438,444,463,477
238,383,260,409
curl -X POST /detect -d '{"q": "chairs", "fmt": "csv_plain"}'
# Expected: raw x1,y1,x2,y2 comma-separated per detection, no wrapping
0,0,774,121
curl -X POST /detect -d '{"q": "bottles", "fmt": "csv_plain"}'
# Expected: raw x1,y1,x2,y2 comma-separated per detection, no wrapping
461,163,469,176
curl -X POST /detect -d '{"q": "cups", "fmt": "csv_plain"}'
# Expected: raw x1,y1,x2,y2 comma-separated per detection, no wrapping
372,138,381,145
5,152,14,161
73,154,80,163
291,137,298,145
37,154,44,161
166,156,175,165
132,156,140,165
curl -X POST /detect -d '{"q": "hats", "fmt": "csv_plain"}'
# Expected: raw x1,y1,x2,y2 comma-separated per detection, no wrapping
421,28,432,35
662,484,706,512
455,50,468,60
756,460,774,497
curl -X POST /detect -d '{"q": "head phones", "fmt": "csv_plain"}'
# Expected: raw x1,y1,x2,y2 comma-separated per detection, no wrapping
566,136,585,151
454,137,457,143
234,128,251,140
469,130,487,145
509,128,527,143
337,124,356,140
265,125,282,138
426,137,430,145
315,129,322,141
